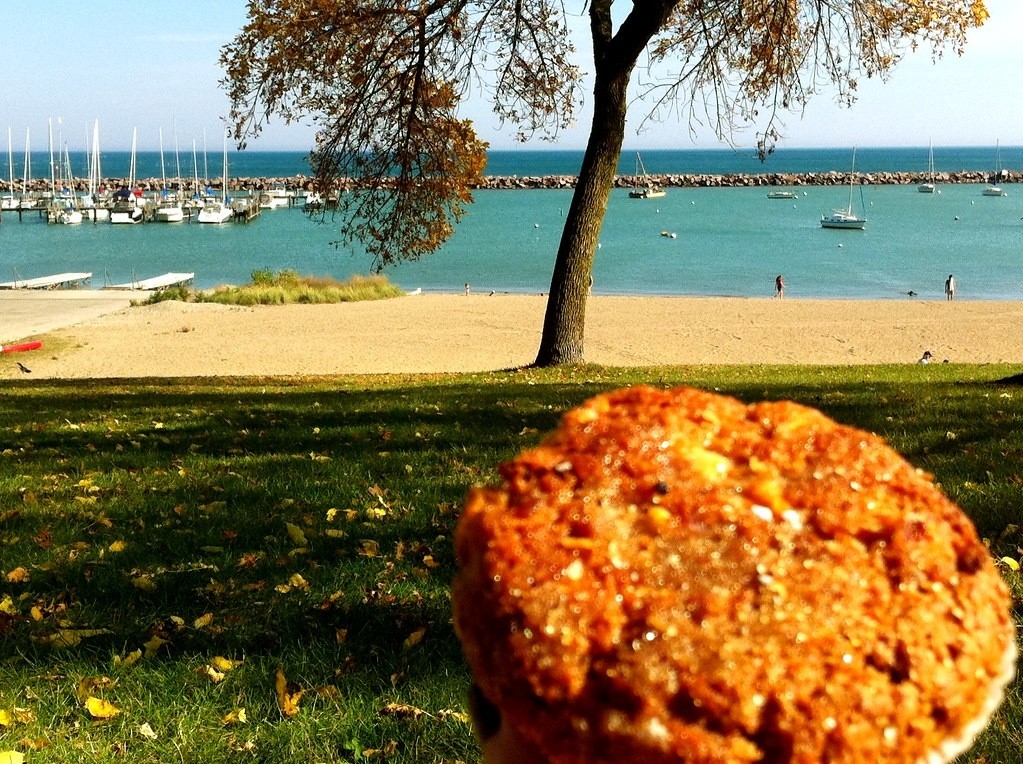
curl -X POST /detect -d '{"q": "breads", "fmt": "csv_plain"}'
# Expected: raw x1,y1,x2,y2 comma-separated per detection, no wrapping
453,384,1018,764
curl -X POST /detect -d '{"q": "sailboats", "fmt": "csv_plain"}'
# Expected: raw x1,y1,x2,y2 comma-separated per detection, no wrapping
628,152,665,198
981,139,1004,196
820,147,867,228
918,140,935,193
198,125,234,224
0,116,210,225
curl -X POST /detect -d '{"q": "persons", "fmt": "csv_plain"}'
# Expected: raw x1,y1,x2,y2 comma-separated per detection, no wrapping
588,273,592,295
943,359,949,363
465,283,469,296
944,274,956,301
771,274,785,299
918,351,933,364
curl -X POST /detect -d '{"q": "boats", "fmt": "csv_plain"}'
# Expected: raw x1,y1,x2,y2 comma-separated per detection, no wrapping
259,192,279,209
306,192,323,210
767,191,793,199
267,181,294,203
